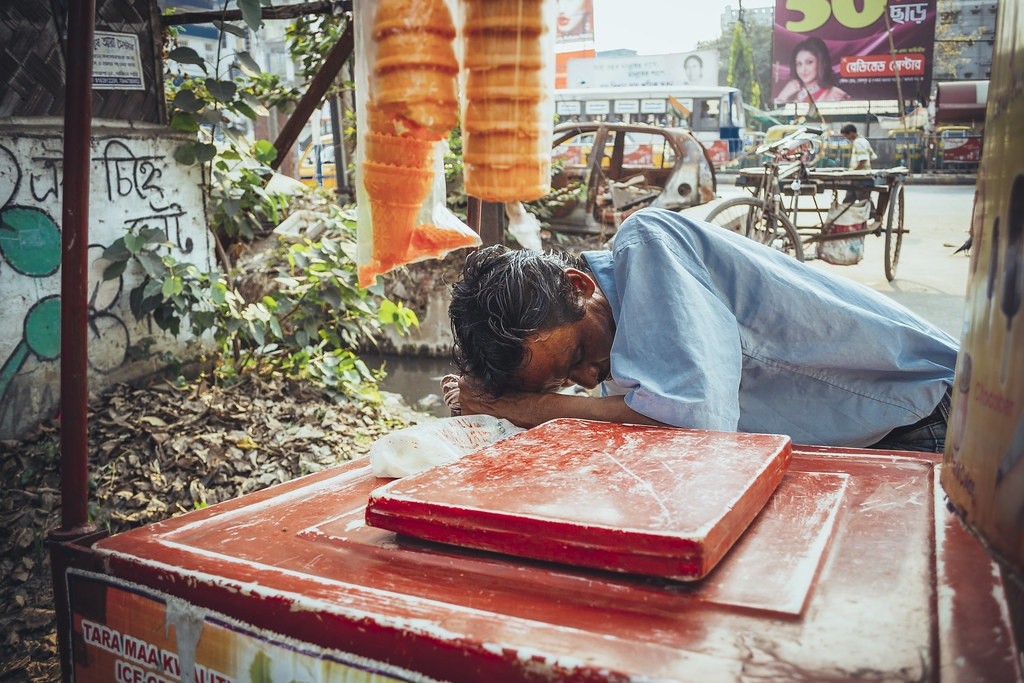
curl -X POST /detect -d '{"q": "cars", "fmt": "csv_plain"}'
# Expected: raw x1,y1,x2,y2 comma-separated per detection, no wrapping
503,122,716,250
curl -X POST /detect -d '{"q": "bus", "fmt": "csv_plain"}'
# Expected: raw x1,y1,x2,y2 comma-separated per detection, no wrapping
554,87,745,170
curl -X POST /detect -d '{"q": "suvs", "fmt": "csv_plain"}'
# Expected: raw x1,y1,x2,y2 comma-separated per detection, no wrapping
931,126,975,170
766,126,852,166
888,130,926,171
298,134,338,195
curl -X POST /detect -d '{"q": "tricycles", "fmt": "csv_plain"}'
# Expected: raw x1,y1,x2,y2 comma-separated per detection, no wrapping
705,128,909,284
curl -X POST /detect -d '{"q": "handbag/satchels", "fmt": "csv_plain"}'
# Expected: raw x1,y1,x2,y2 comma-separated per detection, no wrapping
817,199,871,266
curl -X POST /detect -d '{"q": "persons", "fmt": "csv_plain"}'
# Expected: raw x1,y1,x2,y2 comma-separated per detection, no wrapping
682,55,703,86
448,206,960,453
557,0,586,35
840,124,878,217
774,36,850,103
